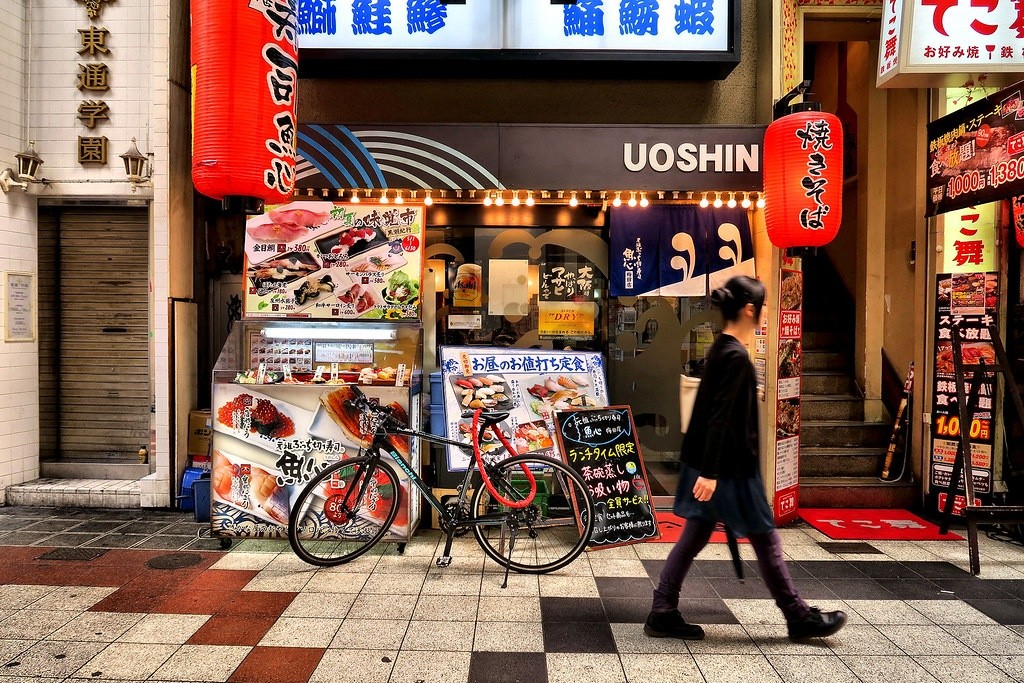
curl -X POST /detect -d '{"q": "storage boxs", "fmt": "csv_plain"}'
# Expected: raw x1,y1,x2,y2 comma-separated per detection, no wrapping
186,409,214,457
179,468,210,511
500,479,549,516
429,488,488,532
190,478,210,523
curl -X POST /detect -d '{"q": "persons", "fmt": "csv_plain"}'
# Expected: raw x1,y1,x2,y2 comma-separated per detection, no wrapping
643,274,846,641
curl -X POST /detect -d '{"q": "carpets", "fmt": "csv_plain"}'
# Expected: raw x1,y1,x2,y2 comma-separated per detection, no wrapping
643,511,750,544
798,507,965,540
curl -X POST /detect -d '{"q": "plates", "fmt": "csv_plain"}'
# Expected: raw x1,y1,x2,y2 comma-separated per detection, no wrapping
520,373,596,422
458,418,512,457
213,384,410,538
228,372,283,384
245,202,409,319
449,374,513,413
514,419,553,453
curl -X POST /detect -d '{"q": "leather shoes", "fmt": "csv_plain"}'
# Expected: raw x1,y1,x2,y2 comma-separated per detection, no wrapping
644,608,705,640
788,606,847,642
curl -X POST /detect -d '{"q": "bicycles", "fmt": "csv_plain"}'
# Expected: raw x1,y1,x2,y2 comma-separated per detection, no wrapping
287,384,596,590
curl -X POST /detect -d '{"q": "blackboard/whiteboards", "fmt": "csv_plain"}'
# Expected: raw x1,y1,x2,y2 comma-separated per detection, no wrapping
552,404,662,552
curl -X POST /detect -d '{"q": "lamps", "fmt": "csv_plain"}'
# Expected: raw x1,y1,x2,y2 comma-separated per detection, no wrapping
0,140,45,192
119,136,153,193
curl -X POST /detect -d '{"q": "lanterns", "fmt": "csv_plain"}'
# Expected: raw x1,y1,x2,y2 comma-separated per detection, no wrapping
191,2,299,217
763,101,844,258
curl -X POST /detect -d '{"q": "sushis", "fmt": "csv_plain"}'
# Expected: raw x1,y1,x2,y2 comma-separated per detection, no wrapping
246,210,392,313
455,374,594,450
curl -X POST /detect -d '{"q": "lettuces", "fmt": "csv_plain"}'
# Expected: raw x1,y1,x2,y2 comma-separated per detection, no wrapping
385,269,420,305
